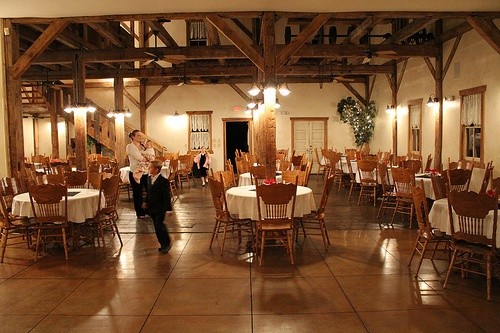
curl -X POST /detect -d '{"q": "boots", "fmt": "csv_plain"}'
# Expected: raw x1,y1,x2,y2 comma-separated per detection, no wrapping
201,176,205,186
205,176,209,182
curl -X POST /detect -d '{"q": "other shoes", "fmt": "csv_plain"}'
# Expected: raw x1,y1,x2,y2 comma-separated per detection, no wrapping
140,215,144,219
158,243,171,252
145,214,150,218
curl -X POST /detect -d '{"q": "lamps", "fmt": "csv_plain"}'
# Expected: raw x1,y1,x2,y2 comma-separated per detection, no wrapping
246,81,290,114
64,99,97,114
425,94,438,104
386,103,394,111
107,106,131,118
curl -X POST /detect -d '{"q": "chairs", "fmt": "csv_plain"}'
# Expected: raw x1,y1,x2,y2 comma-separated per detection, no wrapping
0,149,500,301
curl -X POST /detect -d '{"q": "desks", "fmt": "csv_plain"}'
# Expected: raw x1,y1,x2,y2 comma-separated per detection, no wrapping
338,159,357,173
429,198,500,279
355,165,399,185
224,185,317,251
120,164,170,203
239,170,282,187
392,174,441,201
12,188,106,252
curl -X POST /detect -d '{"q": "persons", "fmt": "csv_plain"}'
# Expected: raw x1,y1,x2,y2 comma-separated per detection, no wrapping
126,129,156,218
194,146,211,185
142,159,172,254
